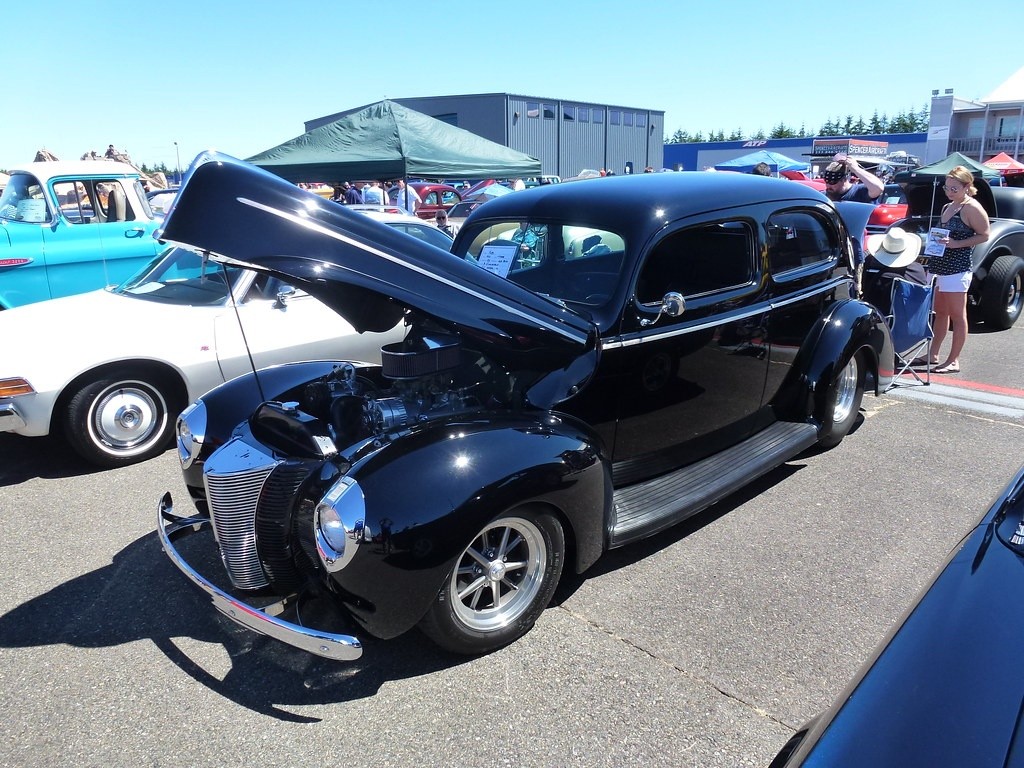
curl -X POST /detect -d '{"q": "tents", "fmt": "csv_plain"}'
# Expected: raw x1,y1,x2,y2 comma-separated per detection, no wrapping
984,152,1024,176
245,99,542,233
895,151,1002,217
715,150,811,180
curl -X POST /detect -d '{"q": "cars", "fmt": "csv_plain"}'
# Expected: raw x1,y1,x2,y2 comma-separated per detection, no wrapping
777,170,1024,328
1,160,235,314
0,200,494,468
768,461,1024,768
305,175,626,273
154,149,895,666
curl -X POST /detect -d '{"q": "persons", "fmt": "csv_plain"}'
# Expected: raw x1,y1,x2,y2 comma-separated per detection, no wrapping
821,156,885,203
644,167,653,173
862,226,928,329
907,166,990,373
434,209,456,240
105,145,115,159
395,179,422,217
328,182,390,212
753,162,767,175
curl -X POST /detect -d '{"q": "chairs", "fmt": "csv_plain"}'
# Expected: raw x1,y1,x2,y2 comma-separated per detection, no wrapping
107,188,126,221
884,273,939,391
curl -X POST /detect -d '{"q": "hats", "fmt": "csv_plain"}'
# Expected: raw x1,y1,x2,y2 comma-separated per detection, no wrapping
867,227,921,267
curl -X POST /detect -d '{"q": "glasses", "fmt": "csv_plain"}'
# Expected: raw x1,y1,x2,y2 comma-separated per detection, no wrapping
943,185,965,193
436,216,447,220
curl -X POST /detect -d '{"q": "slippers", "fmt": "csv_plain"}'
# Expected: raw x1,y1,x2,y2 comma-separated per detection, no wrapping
907,358,938,365
930,365,960,373
